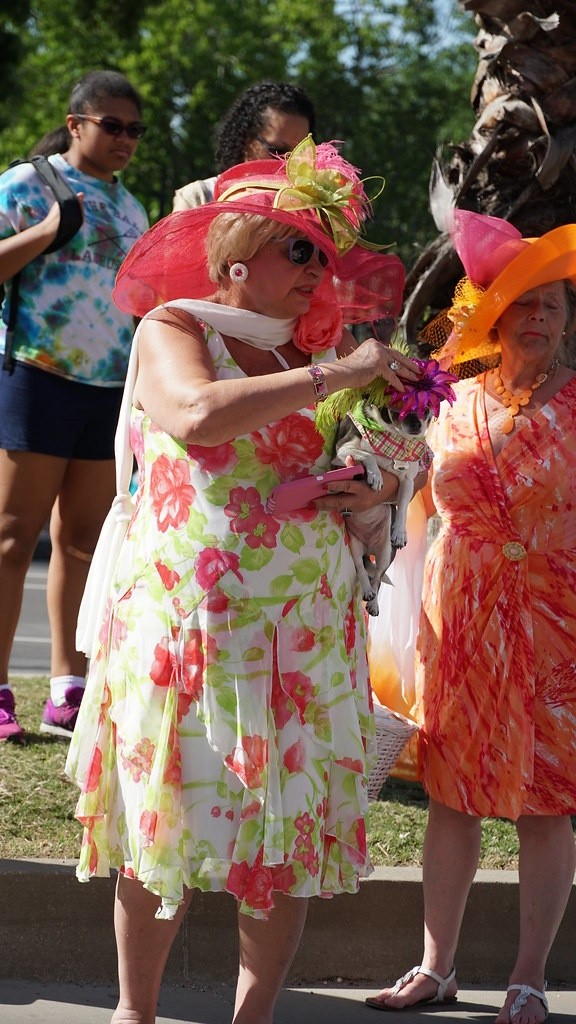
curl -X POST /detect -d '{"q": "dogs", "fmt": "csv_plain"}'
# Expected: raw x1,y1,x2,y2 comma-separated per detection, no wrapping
332,393,432,618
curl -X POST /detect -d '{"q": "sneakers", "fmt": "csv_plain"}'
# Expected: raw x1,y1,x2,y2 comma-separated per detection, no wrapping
39,686,85,737
0,688,26,744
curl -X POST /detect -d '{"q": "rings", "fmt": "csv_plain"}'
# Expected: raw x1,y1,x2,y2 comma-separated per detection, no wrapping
390,360,398,370
339,507,353,517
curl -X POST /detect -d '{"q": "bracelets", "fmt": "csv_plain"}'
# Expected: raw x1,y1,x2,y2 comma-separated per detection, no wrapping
306,362,328,405
381,496,398,506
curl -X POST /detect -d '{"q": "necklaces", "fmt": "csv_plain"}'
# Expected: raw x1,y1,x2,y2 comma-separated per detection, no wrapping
494,359,558,435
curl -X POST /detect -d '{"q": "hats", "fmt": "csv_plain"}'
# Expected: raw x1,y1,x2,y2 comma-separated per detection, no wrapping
417,210,576,379
112,160,406,325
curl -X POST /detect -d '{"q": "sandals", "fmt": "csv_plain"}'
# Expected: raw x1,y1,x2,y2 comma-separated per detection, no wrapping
495,979,548,1024
366,965,457,1011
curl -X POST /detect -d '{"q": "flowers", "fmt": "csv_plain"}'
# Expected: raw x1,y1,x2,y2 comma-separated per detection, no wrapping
272,133,374,232
364,340,458,424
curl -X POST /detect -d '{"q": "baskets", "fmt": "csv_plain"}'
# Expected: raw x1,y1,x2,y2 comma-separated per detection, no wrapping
358,696,421,801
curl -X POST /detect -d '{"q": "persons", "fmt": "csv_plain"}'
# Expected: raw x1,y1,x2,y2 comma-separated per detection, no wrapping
170,75,358,357
64,141,432,1024
365,214,576,1024
0,66,149,737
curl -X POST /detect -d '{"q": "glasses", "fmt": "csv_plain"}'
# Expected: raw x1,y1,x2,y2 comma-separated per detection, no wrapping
265,237,330,270
75,114,144,138
251,134,289,160
